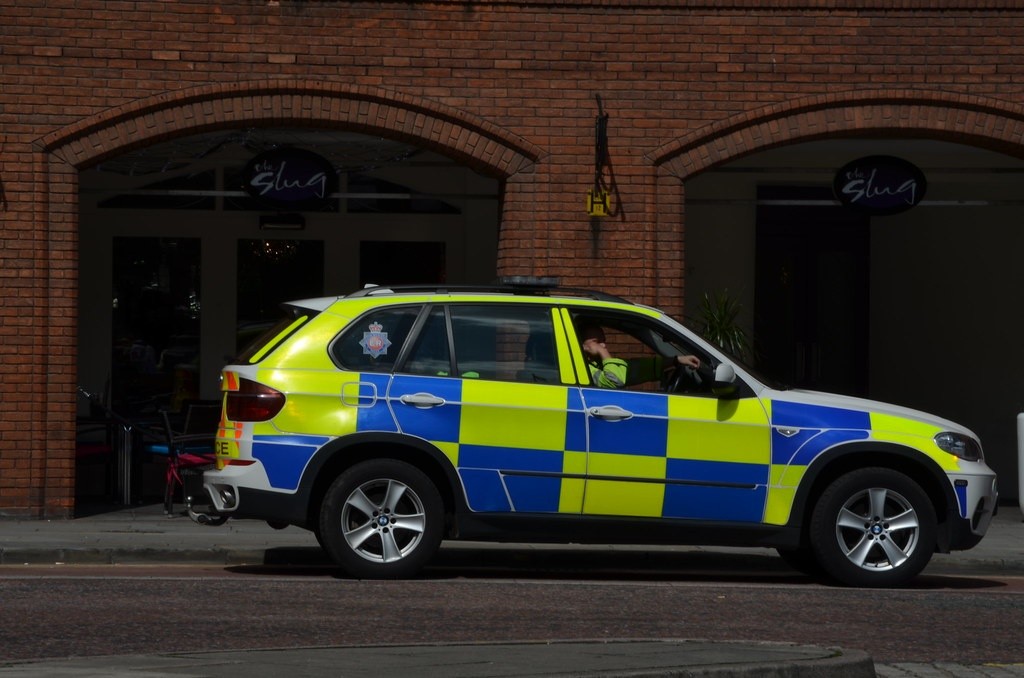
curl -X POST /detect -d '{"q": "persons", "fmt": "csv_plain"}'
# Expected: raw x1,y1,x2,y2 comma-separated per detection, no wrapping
579,324,700,390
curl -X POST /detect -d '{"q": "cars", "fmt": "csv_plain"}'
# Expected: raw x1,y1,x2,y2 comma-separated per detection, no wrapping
183,274,999,587
